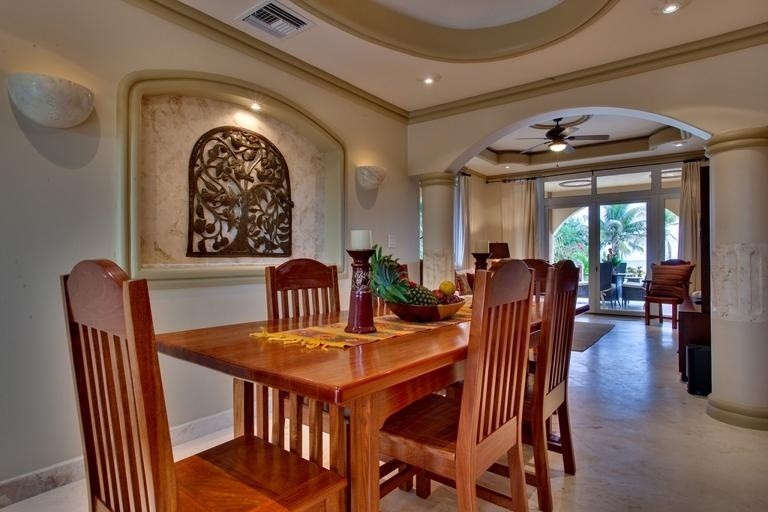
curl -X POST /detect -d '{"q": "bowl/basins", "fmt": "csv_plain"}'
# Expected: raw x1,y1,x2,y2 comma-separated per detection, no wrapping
384,300,467,323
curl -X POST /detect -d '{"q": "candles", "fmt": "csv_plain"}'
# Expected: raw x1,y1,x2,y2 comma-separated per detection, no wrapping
475,240,489,253
351,229,371,249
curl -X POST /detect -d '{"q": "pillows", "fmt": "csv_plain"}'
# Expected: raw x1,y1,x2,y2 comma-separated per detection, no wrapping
650,263,696,299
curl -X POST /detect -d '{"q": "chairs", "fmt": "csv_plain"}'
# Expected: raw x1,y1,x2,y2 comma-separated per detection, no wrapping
600,263,617,309
522,259,557,437
372,264,409,317
59,259,349,512
265,258,413,492
618,263,627,284
446,260,580,512
642,259,692,329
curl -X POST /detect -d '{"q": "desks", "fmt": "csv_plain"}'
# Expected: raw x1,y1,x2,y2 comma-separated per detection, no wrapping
154,295,590,512
622,282,646,307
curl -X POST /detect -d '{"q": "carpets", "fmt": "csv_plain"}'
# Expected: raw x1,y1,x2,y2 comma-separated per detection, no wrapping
576,303,589,316
572,322,615,351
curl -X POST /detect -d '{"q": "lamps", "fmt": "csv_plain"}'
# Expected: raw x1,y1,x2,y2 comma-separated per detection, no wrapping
548,141,568,152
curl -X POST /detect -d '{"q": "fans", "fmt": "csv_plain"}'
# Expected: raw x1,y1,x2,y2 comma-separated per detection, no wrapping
517,118,609,154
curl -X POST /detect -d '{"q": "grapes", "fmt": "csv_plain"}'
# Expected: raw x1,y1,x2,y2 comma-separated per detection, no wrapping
439,295,459,304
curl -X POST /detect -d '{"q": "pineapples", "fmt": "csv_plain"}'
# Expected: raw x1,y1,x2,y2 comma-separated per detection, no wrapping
408,285,438,306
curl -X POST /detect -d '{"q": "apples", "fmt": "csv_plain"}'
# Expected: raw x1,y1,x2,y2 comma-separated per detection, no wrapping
432,289,444,299
